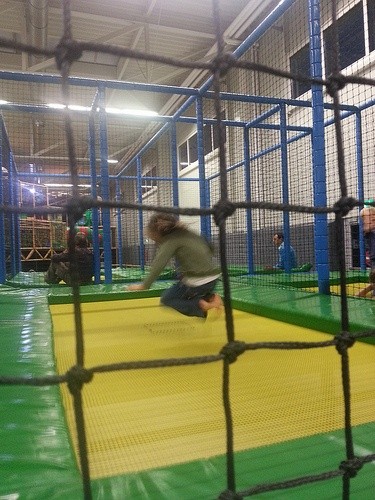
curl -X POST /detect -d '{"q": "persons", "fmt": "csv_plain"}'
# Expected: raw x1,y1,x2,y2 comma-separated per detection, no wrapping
263,232,298,271
356,207,375,298
43,231,94,287
126,213,222,318
